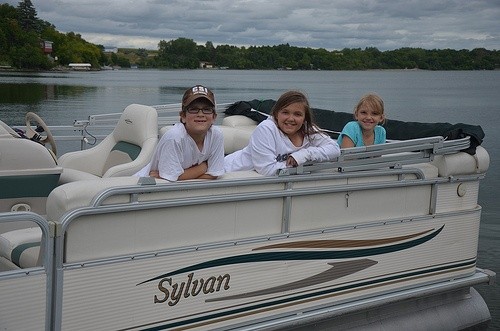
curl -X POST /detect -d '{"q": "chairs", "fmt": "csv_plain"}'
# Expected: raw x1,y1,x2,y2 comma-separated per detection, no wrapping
58,104,158,184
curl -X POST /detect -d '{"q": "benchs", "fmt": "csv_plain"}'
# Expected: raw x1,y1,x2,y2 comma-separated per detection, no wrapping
0,99,489,272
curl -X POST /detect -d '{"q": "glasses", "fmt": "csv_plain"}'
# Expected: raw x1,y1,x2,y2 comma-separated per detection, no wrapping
186,106,214,114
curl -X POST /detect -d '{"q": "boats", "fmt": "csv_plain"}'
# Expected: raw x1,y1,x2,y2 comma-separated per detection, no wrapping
69,63,91,71
0,99,497,331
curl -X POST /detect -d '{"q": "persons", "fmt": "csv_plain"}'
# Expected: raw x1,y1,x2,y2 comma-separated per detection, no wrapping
223,91,340,177
132,84,224,181
336,94,386,150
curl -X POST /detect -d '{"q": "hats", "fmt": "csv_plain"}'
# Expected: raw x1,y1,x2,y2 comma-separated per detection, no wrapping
181,84,215,110
